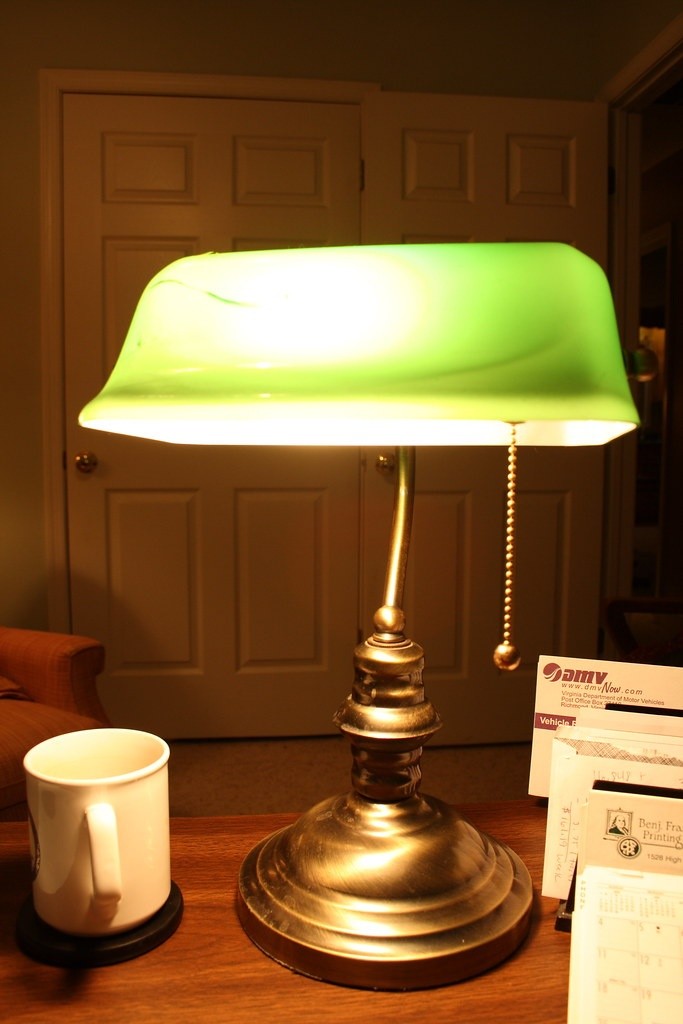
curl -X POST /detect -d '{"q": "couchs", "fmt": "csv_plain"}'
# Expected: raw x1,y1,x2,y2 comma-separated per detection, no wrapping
0,624,116,820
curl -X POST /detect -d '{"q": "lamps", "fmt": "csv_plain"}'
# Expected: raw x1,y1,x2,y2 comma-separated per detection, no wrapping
76,240,637,990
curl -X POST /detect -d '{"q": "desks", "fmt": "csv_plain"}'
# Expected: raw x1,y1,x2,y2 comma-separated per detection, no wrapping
0,798,571,1024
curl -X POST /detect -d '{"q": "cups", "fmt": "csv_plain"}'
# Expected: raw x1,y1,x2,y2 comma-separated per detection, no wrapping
22,727,172,937
